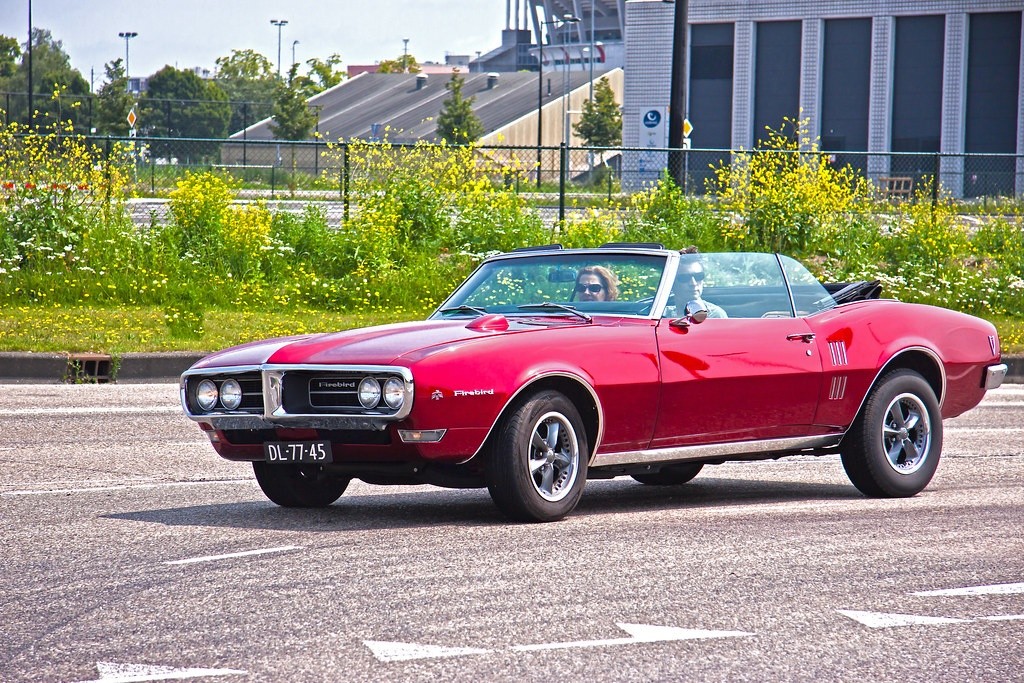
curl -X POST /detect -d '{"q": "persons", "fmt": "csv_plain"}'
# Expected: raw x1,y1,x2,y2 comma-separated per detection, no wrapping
672,245,728,319
576,265,618,301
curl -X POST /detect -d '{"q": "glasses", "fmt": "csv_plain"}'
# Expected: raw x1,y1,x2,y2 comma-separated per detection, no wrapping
678,272,704,283
576,284,606,292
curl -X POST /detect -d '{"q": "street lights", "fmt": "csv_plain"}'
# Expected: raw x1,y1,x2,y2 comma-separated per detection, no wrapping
119,32,138,91
271,19,289,78
291,40,300,65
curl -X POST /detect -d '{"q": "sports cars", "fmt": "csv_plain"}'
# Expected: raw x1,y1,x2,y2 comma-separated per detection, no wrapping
180,243,1002,525
